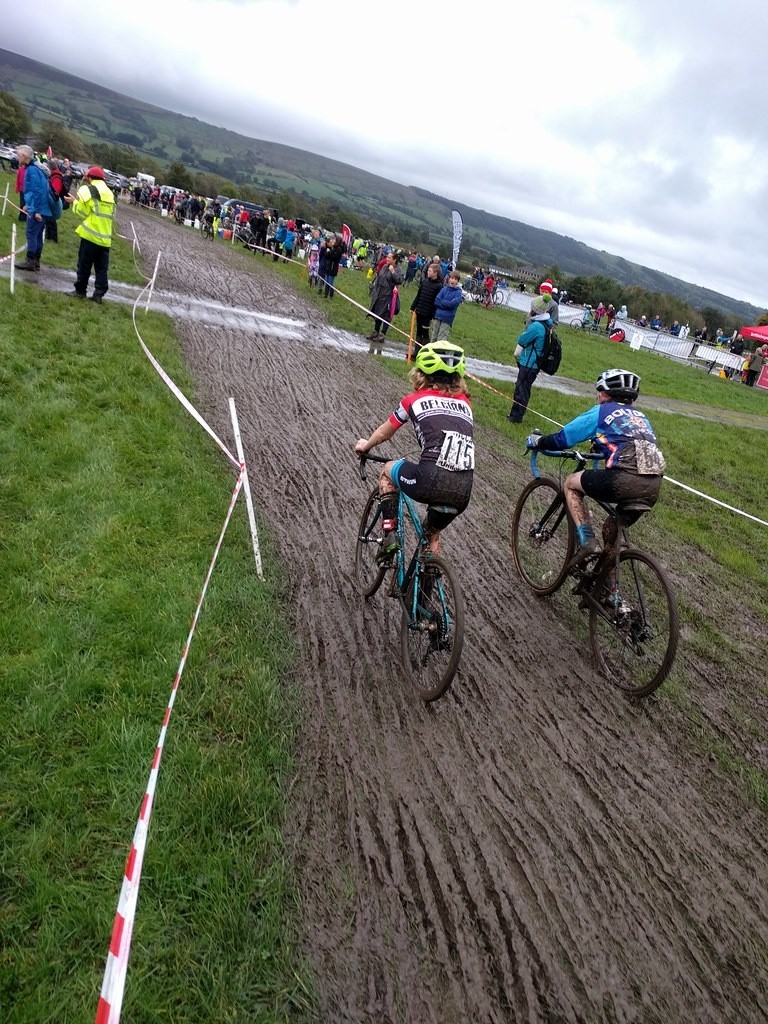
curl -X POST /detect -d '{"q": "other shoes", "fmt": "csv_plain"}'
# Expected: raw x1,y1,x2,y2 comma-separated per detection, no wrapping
317,289,322,295
373,334,385,343
91,294,103,304
63,289,84,299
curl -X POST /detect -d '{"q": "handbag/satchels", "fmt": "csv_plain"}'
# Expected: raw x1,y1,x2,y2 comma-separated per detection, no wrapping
388,294,401,314
46,182,63,218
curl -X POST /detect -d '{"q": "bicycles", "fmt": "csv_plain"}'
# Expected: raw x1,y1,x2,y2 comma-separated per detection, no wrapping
569,315,602,337
510,425,678,697
462,277,503,306
352,449,466,705
198,218,214,242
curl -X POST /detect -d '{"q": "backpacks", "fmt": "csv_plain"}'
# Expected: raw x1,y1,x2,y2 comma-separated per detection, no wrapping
533,322,562,375
52,174,70,210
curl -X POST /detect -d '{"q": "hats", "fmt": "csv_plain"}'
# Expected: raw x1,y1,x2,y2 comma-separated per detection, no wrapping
539,279,552,293
531,293,552,314
88,166,104,179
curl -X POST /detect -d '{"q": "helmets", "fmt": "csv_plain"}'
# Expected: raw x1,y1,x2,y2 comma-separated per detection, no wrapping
416,340,465,378
597,368,641,400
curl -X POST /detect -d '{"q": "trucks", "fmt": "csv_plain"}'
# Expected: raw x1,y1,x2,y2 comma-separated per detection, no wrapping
137,172,155,190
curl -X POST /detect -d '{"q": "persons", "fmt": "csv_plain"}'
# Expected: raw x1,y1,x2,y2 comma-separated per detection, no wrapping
0,139,570,425
526,369,667,606
583,302,768,386
355,340,475,614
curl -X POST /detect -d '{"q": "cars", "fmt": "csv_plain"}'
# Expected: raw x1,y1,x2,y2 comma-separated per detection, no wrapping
0,146,19,161
70,161,131,190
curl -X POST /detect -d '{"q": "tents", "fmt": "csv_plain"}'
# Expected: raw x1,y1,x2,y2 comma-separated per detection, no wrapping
739,324,768,344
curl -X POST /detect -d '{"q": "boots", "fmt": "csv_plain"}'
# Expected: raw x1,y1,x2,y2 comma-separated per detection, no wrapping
36,256,40,270
15,257,36,271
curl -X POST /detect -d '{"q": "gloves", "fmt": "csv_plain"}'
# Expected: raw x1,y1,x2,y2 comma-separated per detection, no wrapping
526,433,541,450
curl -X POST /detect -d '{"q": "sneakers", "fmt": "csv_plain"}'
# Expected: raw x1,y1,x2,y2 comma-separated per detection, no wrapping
568,538,601,569
604,580,621,606
411,613,427,630
375,530,399,562
366,331,379,339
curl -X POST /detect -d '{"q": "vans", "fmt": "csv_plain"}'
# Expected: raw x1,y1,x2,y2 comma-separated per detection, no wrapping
160,184,183,197
222,198,278,224
